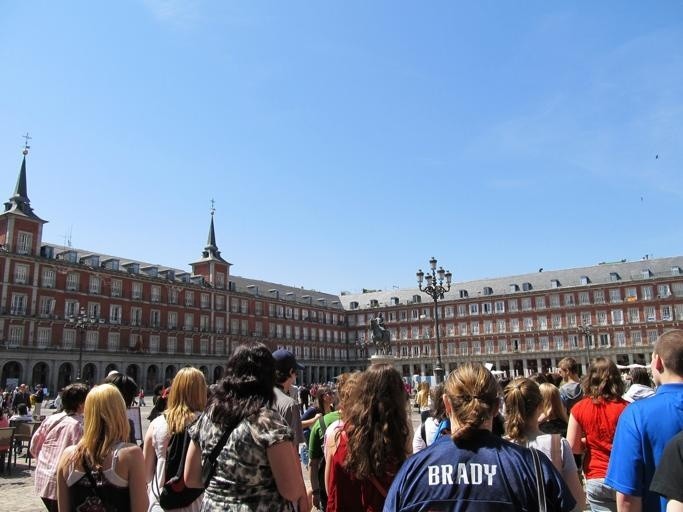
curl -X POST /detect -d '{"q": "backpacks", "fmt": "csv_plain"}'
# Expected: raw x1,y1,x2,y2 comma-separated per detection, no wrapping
159,410,214,511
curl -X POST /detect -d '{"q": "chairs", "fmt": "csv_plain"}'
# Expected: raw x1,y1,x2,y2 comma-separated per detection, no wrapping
0,419,35,475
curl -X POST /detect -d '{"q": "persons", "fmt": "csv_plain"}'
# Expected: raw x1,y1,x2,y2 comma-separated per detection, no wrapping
1,326,683,512
374,312,386,338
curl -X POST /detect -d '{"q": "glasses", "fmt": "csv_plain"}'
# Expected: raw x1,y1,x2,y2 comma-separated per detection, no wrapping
323,391,335,397
559,370,568,373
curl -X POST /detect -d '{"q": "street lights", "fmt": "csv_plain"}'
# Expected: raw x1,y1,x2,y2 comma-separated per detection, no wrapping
67,305,96,383
415,254,451,384
576,319,592,368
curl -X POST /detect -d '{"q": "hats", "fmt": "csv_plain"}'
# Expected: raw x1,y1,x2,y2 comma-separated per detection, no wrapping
17,403,28,416
273,349,305,370
